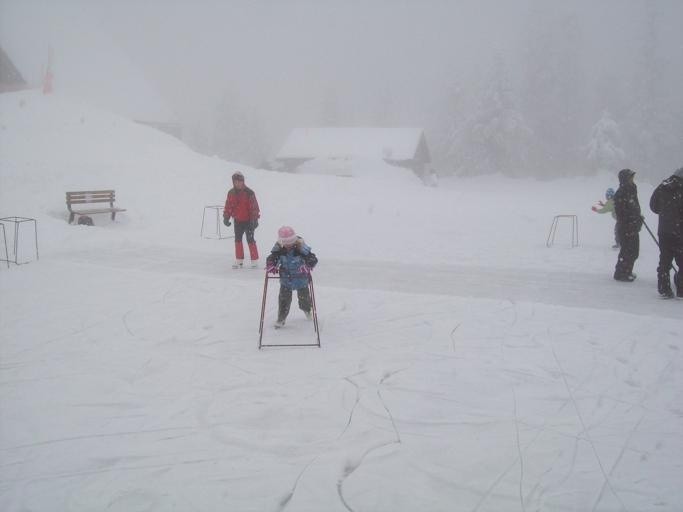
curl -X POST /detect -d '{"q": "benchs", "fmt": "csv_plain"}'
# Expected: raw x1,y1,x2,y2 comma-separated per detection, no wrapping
66,190,126,223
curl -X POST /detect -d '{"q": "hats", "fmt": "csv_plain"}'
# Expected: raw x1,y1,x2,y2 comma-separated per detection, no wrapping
605,169,636,199
231,171,245,182
277,226,298,243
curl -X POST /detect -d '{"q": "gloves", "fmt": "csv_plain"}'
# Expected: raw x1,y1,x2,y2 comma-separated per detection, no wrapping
249,219,260,230
222,216,232,227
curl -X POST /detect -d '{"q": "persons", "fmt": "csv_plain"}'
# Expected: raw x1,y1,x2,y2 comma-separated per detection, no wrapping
223,171,261,270
613,168,643,283
266,225,318,328
591,187,619,249
650,166,683,299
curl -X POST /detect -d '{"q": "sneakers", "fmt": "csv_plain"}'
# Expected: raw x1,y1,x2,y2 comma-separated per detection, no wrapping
272,309,315,329
231,258,260,267
